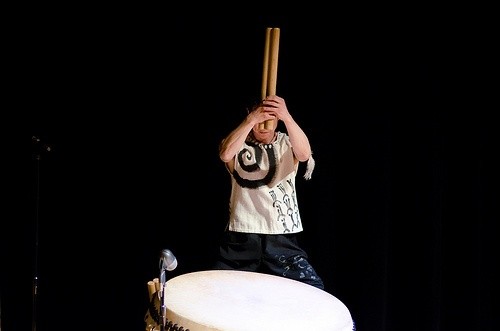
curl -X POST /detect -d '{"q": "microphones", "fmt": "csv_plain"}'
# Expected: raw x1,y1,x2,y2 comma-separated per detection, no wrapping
162,249,178,271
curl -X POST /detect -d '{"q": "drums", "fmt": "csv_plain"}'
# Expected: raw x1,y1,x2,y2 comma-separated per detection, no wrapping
143,268,358,331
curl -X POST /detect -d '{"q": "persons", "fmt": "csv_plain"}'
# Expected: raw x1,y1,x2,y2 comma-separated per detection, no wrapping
208,95,324,289
